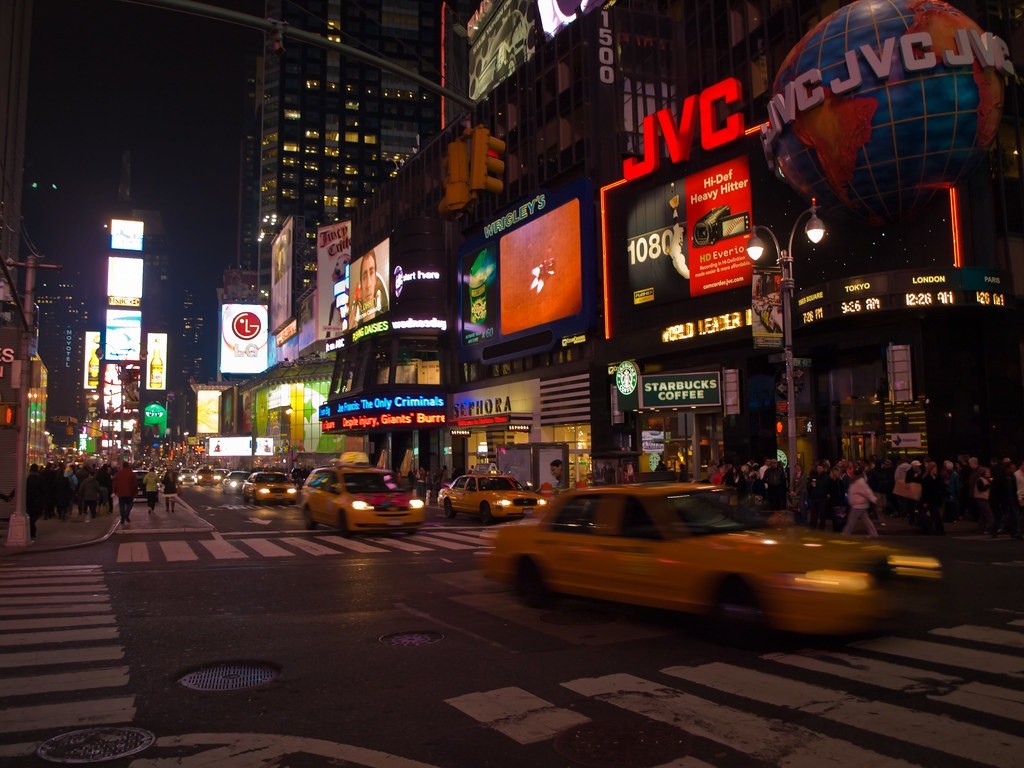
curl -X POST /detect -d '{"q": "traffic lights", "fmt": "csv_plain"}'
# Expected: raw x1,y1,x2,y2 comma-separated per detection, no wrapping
470,129,505,194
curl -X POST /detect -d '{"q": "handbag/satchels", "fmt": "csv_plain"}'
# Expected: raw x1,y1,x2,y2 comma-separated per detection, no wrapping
788,494,801,512
893,479,923,500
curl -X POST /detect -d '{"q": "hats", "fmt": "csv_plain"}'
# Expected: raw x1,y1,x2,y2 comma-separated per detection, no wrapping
911,460,921,466
1003,457,1010,463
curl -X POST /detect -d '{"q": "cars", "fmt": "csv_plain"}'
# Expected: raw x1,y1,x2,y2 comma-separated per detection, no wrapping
132,470,159,503
222,471,251,495
242,472,298,505
155,466,230,487
438,463,548,524
301,452,426,539
473,482,898,649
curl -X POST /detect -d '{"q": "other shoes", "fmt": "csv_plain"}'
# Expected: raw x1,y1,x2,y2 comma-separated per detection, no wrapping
93,513,96,518
121,519,125,524
126,515,130,522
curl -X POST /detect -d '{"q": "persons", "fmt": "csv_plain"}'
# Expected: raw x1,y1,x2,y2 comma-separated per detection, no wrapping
654,456,1024,541
0,462,183,540
550,459,562,488
237,464,474,498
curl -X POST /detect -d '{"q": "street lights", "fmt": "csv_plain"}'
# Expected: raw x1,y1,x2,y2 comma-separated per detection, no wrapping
3,257,63,548
746,198,825,510
